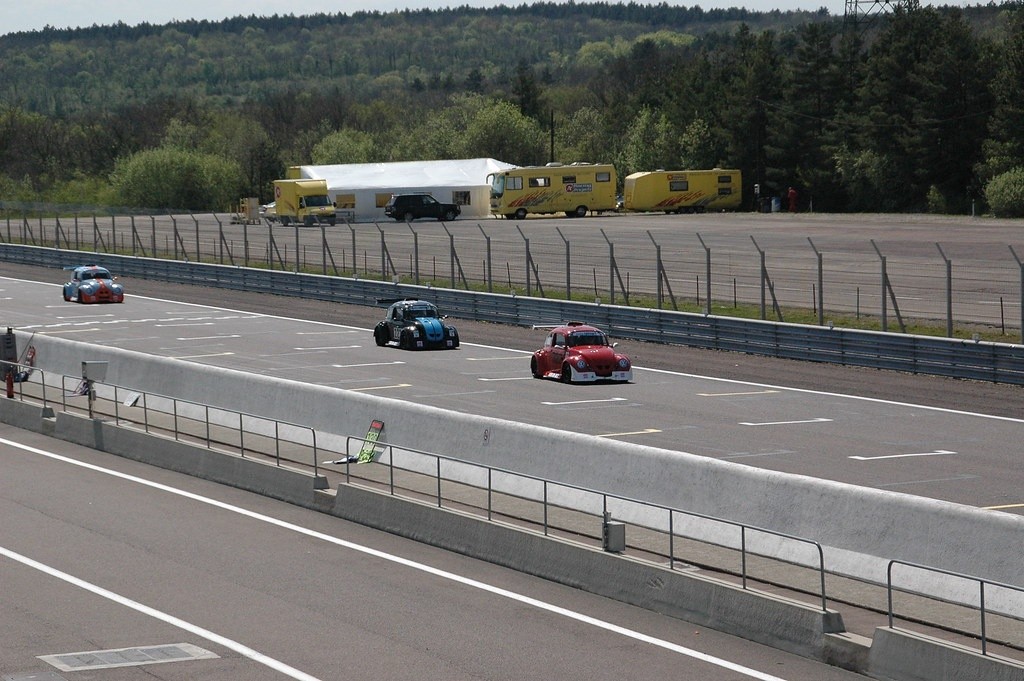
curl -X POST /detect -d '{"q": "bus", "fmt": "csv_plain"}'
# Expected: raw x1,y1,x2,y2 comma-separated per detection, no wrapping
486,162,620,221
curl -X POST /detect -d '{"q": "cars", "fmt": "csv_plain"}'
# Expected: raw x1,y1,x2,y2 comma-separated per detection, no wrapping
262,201,276,222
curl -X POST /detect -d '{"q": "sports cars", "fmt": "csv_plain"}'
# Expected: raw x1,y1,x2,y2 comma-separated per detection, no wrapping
374,299,460,352
62,263,125,304
530,322,634,383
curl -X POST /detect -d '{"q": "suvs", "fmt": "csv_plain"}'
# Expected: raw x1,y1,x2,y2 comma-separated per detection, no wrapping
384,193,462,223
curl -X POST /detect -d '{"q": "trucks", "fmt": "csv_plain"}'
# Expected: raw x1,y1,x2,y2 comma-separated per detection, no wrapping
273,179,338,228
